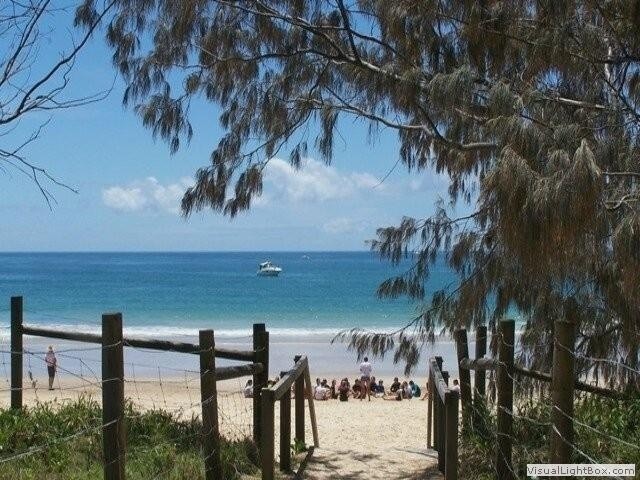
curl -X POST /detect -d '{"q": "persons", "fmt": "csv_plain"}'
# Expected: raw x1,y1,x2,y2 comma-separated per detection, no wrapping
44,345,57,390
243,356,421,401
451,380,461,399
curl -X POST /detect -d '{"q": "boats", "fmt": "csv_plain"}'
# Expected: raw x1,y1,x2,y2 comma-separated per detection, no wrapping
257,261,281,276
